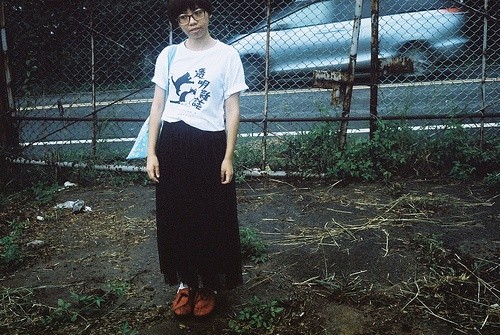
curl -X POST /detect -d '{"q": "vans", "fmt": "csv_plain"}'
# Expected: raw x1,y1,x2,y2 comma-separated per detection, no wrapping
228,1,470,90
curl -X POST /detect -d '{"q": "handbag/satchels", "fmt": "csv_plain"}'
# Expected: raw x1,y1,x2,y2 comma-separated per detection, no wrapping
126,45,177,159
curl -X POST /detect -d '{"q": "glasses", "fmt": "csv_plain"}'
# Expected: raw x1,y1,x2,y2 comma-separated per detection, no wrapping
175,8,206,25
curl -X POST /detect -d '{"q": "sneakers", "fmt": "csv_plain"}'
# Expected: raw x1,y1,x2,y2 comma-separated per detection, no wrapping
193,288,217,319
173,287,193,317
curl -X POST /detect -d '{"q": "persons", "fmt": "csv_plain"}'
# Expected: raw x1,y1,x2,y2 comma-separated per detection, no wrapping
146,0,249,317
